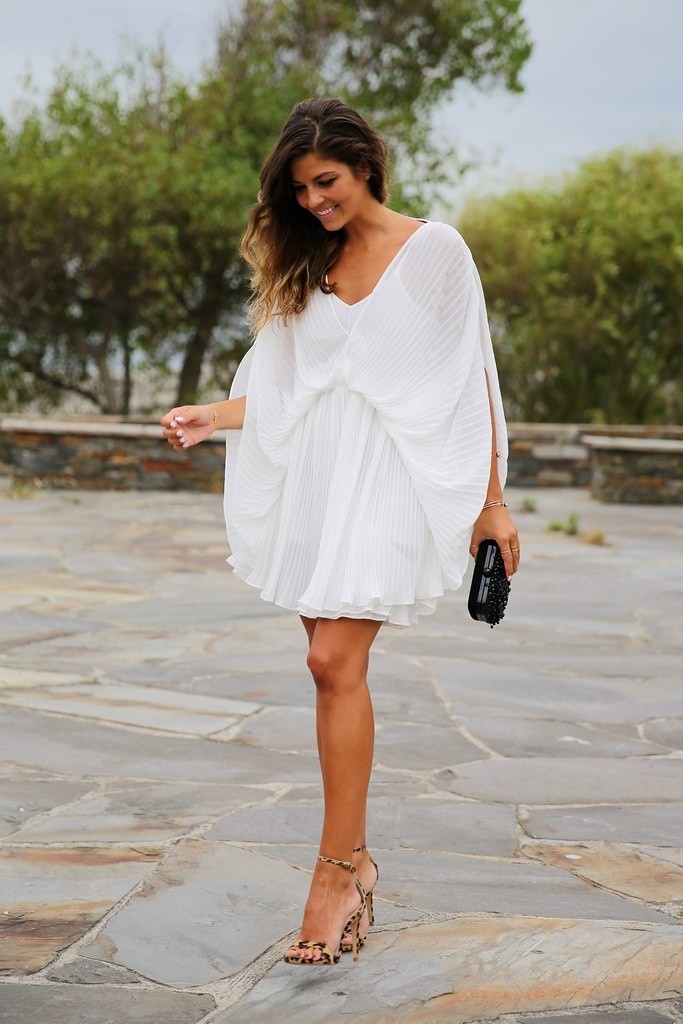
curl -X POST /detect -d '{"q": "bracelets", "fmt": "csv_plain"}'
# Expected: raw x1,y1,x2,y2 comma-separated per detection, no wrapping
482,501,507,508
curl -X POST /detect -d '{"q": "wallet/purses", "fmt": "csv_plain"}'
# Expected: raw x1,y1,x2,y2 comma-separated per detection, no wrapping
467,539,512,629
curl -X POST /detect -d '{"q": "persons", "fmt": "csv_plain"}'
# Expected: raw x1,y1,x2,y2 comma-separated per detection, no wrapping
158,97,521,964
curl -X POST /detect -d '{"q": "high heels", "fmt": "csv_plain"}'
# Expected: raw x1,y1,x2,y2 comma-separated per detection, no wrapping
283,854,366,965
340,844,380,952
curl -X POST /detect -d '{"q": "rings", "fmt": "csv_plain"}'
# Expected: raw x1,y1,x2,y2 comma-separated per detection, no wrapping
469,545,478,548
500,549,511,554
511,545,518,551
